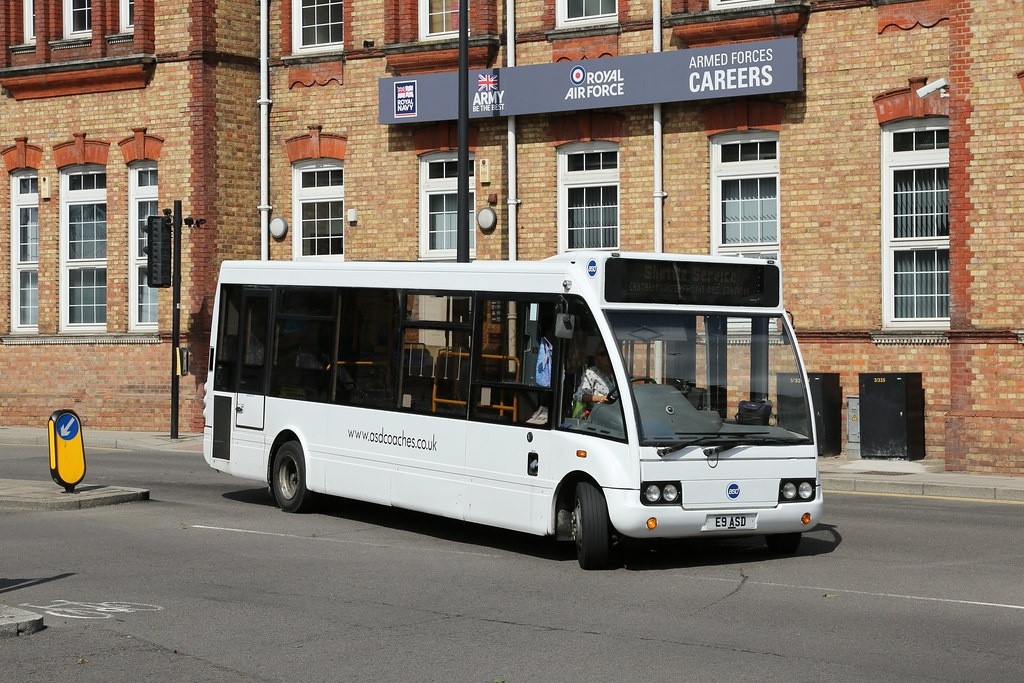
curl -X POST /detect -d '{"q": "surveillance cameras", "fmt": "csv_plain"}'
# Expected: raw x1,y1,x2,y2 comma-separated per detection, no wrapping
915,78,948,99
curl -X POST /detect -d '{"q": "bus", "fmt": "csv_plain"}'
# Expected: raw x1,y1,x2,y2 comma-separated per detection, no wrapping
200,248,826,574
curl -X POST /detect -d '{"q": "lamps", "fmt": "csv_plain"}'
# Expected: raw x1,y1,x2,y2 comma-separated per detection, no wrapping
269,216,289,238
477,207,496,231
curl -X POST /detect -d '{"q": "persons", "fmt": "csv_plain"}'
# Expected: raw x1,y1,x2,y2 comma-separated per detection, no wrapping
572,342,620,411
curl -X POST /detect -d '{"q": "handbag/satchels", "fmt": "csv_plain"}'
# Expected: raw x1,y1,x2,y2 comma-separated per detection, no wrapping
536,336,552,388
527,406,548,425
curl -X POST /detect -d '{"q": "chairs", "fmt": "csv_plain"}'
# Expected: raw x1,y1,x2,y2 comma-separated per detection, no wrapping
242,333,501,424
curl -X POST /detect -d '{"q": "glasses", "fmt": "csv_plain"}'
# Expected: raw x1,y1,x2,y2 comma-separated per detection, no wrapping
597,350,608,355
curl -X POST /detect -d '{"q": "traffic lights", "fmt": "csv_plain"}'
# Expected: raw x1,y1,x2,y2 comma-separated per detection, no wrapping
141,215,171,289
